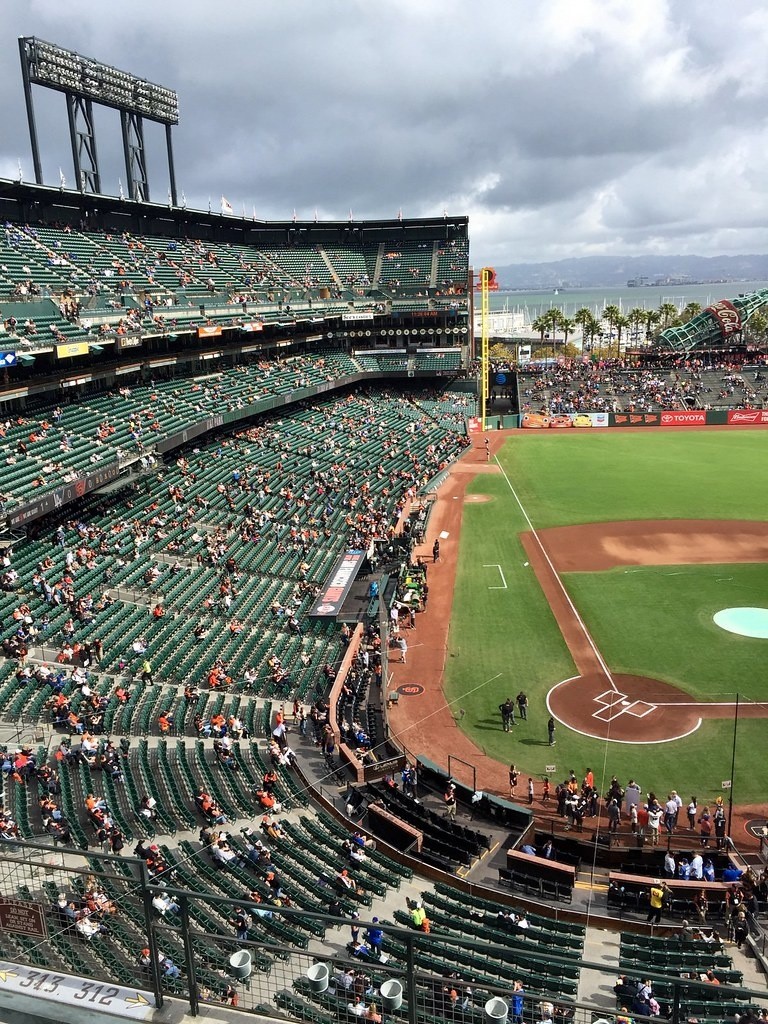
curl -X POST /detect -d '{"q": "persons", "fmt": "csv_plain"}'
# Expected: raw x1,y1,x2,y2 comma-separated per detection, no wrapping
0,228,768,1024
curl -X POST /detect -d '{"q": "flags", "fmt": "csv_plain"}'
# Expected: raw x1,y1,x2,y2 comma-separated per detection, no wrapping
293,209,296,219
315,209,318,219
444,211,448,217
253,207,256,218
221,197,233,213
398,209,403,218
18,161,23,180
60,169,66,187
136,184,143,204
183,194,187,210
168,189,172,211
119,181,124,198
209,199,211,210
350,210,353,219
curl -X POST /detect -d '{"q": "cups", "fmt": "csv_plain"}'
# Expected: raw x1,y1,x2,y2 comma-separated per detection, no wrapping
483,996,509,1024
306,962,329,993
380,979,403,1010
229,949,252,978
